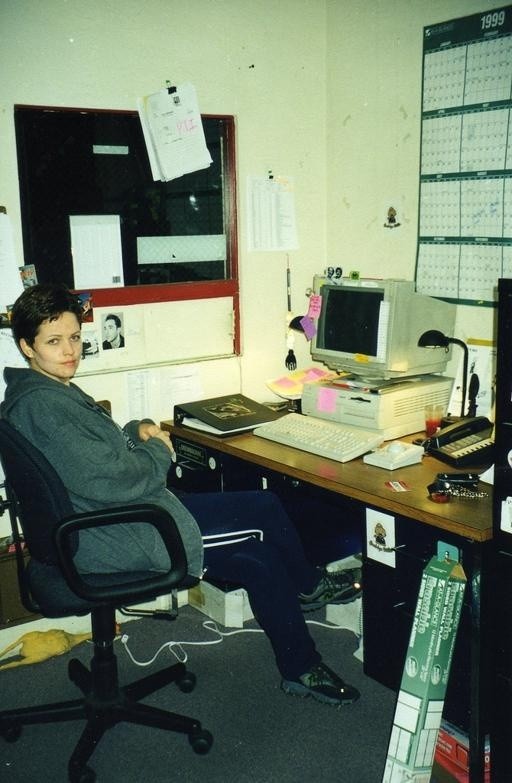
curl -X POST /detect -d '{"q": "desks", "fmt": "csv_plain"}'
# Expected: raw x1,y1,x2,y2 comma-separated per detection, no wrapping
159,401,489,783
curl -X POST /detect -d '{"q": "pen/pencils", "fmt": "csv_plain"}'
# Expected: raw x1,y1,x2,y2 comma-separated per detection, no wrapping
331,383,370,392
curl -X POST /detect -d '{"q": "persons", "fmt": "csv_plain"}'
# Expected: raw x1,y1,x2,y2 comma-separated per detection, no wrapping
335,267,342,279
1,279,363,706
327,266,334,277
102,314,124,349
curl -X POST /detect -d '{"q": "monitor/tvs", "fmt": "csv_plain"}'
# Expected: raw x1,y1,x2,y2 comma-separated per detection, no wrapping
307,274,457,391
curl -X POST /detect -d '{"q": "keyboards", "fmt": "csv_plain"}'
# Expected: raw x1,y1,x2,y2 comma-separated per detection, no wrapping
253,410,383,464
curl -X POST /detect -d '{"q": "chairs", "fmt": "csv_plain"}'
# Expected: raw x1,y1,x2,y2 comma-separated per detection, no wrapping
0,415,216,779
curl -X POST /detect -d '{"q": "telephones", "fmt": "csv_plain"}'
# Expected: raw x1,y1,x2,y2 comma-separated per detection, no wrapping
428,416,495,468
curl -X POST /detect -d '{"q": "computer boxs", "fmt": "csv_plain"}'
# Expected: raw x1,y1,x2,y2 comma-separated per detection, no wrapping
300,373,455,442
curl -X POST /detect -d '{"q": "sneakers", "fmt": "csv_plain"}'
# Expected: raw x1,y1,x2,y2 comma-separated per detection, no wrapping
298,565,364,614
279,659,361,705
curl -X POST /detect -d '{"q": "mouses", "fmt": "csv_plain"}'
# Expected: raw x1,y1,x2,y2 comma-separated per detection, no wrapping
362,439,425,471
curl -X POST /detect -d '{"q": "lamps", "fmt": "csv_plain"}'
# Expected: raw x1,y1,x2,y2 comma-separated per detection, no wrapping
419,331,469,425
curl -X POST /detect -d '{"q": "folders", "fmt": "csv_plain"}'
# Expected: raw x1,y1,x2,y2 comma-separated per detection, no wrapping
174,394,281,438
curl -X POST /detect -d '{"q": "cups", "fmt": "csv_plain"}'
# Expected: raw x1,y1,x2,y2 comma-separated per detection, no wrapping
424,405,444,438
